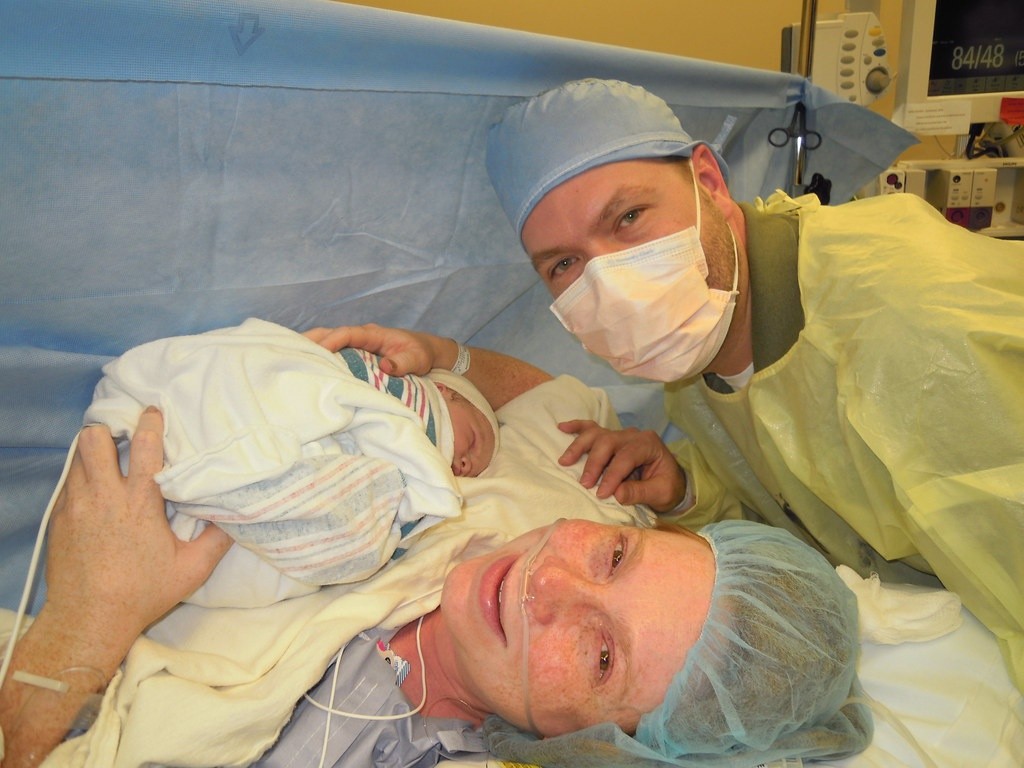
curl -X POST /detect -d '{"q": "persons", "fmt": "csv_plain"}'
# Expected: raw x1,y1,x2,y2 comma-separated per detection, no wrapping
0,322,877,760
89,328,496,585
488,74,1022,686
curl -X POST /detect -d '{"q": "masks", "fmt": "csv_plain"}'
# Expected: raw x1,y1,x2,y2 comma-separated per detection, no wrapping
548,160,741,385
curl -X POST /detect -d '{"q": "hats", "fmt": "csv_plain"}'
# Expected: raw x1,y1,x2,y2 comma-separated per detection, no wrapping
488,78,730,252
428,365,500,465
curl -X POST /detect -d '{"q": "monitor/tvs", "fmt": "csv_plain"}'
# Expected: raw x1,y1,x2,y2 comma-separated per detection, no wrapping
895,0,1024,123
